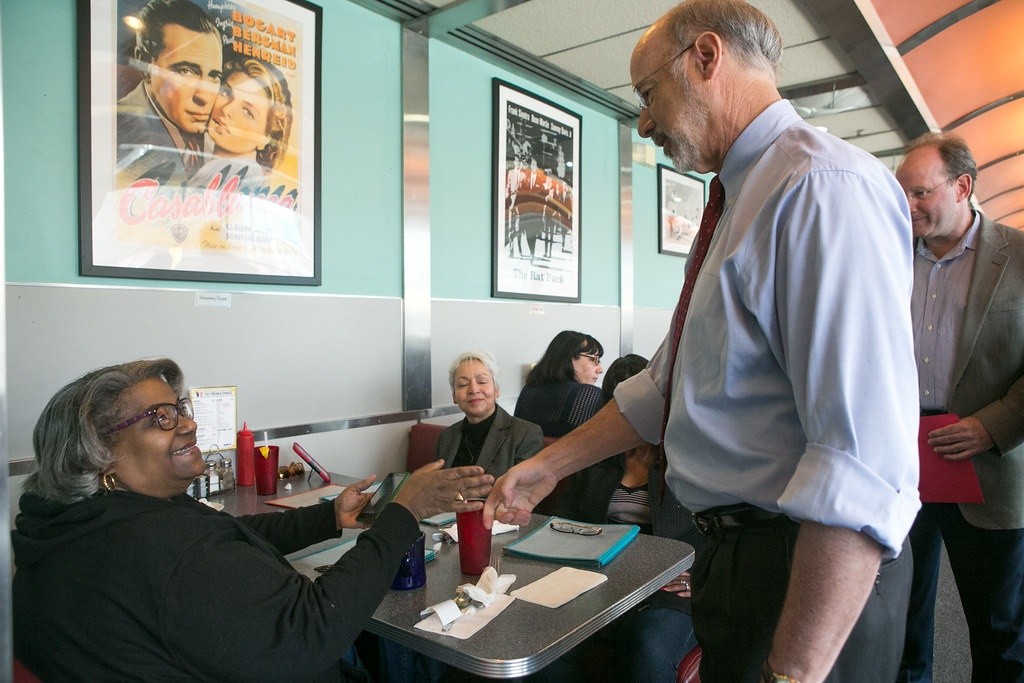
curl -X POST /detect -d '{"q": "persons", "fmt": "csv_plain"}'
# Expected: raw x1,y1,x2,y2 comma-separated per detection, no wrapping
12,357,495,683
433,354,544,502
116,0,292,198
514,331,603,439
897,131,1024,683
483,0,922,683
522,354,698,683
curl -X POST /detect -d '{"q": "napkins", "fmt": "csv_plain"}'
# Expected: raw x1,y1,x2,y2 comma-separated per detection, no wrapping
414,595,515,640
511,567,608,608
438,520,519,543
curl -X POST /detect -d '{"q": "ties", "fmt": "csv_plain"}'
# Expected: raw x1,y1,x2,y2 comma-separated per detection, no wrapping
185,130,204,178
656,174,724,503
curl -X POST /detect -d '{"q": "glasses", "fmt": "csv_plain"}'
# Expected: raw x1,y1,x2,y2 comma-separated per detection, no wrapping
631,43,695,110
905,173,963,204
575,351,602,366
102,398,195,436
550,521,603,537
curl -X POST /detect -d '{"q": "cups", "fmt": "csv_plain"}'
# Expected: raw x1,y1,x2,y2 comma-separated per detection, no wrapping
456,500,492,576
390,531,427,589
254,445,279,496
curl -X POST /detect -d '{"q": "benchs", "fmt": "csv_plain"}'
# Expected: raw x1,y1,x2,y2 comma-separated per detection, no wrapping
407,422,702,683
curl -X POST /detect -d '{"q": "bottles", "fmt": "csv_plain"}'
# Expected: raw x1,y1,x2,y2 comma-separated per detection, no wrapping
203,459,234,496
237,421,254,486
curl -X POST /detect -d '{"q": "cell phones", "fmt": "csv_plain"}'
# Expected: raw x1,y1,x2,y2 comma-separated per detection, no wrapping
356,472,411,523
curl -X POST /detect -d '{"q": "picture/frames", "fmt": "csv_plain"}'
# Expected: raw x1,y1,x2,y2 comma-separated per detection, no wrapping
491,77,583,303
76,0,323,285
658,163,705,257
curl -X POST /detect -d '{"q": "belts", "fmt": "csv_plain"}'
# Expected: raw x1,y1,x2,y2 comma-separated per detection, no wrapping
689,504,780,534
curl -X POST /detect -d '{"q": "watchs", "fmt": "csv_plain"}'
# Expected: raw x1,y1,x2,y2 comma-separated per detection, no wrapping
760,655,801,683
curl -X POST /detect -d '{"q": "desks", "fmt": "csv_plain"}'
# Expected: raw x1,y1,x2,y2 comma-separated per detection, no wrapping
207,472,695,683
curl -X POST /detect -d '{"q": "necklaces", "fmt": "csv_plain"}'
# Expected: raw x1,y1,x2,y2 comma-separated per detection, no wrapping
465,429,481,464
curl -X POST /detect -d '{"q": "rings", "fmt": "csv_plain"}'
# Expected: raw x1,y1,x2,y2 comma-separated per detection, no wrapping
680,580,686,584
456,493,464,501
686,584,690,591
